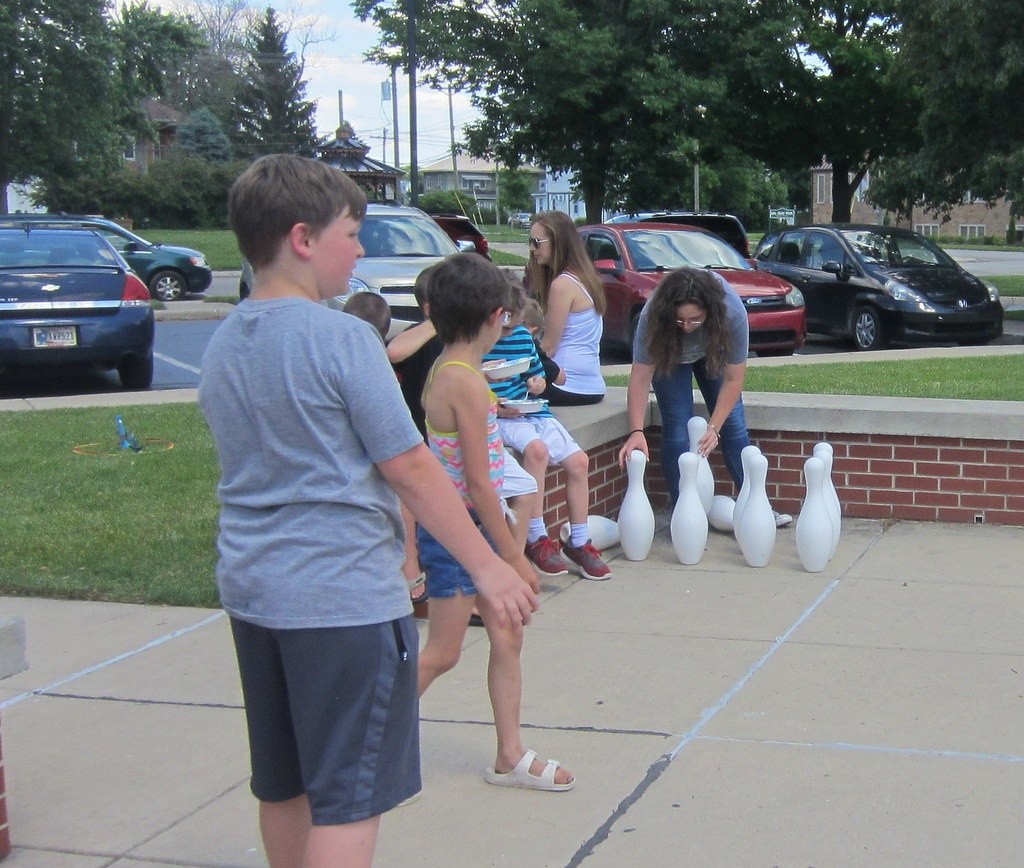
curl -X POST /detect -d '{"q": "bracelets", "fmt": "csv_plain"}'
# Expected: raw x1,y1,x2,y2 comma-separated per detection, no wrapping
629,429,644,435
707,423,721,440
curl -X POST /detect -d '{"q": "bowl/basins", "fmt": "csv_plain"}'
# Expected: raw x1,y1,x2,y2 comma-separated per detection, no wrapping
481,355,535,380
500,399,550,414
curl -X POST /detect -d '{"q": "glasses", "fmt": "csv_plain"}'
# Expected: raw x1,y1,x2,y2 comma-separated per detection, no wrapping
529,237,549,248
675,313,707,327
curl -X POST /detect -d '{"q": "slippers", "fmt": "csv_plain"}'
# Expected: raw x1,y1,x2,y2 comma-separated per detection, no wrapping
484,752,576,793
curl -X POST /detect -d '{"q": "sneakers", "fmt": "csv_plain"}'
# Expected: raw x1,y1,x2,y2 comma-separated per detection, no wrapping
524,534,569,576
772,508,793,528
559,536,612,580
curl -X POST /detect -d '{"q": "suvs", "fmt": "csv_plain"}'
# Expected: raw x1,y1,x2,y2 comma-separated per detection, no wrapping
0,211,213,302
239,203,478,347
427,212,493,264
603,208,750,261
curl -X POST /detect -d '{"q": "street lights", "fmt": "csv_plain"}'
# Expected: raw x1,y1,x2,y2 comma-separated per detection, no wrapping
693,105,708,214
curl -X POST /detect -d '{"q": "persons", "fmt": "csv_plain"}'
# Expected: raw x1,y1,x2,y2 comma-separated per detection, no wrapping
414,251,576,793
341,262,614,626
528,210,608,406
200,155,539,866
619,266,794,528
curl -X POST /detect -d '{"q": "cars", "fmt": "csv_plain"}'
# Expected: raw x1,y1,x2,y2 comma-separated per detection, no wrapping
752,225,1004,351
576,222,808,361
508,213,533,229
0,225,154,392
